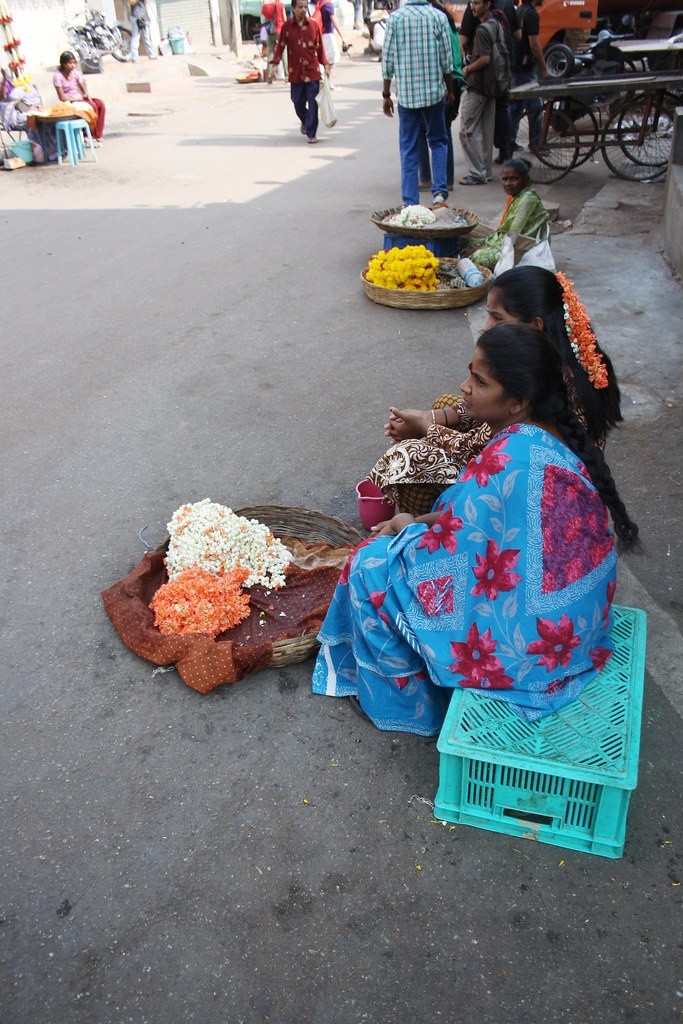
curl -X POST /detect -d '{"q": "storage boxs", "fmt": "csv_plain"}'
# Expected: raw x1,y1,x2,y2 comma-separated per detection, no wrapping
435,606,648,859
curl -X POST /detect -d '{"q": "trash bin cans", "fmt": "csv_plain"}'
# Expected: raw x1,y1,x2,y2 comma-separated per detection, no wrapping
168,37,184,55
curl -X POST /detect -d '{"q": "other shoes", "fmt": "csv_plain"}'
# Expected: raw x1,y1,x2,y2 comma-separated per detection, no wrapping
417,180,433,190
433,195,444,204
307,136,317,144
300,122,307,135
446,185,453,191
513,146,523,153
531,146,552,155
495,155,510,164
459,175,492,185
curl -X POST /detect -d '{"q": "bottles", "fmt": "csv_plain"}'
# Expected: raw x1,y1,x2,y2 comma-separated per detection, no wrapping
457,257,485,287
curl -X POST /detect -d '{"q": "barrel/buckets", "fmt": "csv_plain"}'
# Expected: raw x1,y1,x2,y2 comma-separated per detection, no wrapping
169,37,185,54
356,479,395,530
10,140,34,164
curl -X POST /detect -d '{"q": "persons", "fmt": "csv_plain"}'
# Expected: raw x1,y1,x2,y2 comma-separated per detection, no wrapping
347,0,662,206
313,265,644,740
260,0,346,144
0,51,105,161
459,156,551,274
123,0,158,64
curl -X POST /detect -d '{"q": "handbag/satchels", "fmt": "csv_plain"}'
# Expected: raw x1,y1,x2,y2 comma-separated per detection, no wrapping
136,17,146,29
262,19,276,35
315,74,337,127
492,223,556,279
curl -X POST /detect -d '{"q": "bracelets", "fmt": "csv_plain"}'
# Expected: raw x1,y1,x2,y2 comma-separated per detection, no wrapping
442,408,448,426
432,410,436,424
464,66,469,73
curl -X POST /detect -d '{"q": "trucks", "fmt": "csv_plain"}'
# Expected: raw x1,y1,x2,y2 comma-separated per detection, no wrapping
441,0,683,79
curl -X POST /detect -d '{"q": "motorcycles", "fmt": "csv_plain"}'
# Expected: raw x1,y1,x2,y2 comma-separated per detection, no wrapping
65,1,135,71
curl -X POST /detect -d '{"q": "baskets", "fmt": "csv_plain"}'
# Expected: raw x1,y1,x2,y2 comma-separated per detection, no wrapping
143,505,367,669
360,256,492,310
370,203,479,238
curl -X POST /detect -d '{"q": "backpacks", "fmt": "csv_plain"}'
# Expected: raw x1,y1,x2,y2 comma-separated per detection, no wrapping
311,0,330,34
481,22,512,97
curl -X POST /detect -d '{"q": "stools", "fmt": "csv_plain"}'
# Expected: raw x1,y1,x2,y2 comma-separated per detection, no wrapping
55,118,97,166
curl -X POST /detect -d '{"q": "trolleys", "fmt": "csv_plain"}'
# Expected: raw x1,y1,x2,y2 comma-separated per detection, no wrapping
509,70,683,184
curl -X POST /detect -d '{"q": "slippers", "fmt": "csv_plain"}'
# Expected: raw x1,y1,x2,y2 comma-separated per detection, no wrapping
350,693,373,722
